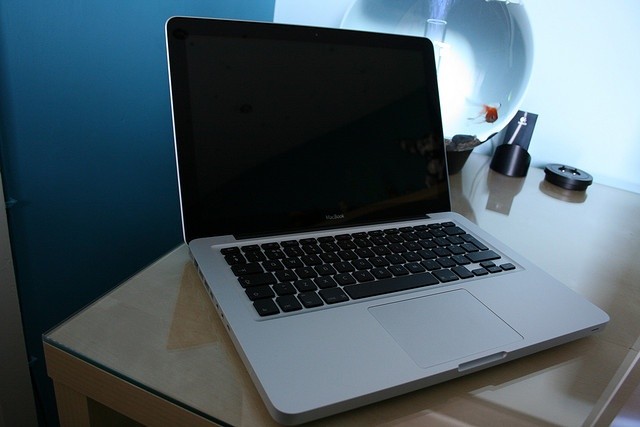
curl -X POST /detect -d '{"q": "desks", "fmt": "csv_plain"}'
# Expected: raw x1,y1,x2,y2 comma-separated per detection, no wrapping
40,150,636,425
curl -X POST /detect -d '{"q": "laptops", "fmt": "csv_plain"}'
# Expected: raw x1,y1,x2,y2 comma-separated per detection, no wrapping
164,14,611,426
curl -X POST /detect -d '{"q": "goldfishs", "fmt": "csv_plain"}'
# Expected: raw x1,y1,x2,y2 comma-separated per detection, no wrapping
467,102,502,124
